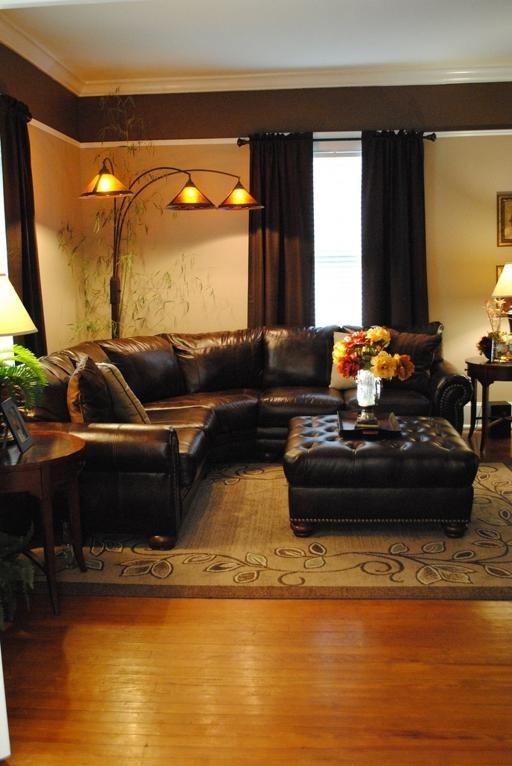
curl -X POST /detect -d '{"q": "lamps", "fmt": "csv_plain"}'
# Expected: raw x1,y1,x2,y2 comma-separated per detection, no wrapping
0,274,38,336
492,263,512,334
79,157,264,338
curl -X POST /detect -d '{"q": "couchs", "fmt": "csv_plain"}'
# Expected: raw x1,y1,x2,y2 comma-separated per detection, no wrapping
7,322,475,548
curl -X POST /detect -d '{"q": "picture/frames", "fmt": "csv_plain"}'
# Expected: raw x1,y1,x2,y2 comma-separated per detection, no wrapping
497,191,512,247
0,398,34,454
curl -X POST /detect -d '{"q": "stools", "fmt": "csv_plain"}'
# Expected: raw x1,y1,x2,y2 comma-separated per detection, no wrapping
283,414,478,539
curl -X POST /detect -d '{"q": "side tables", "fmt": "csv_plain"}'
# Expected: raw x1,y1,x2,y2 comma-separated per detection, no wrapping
465,356,512,462
0,432,86,616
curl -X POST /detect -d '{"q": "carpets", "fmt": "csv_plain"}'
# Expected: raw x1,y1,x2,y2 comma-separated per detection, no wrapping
0,452,512,597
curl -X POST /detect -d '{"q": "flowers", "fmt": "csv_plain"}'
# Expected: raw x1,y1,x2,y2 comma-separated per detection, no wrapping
332,323,415,382
476,297,512,364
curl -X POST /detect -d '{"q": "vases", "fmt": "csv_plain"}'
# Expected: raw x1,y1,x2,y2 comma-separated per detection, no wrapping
354,370,381,423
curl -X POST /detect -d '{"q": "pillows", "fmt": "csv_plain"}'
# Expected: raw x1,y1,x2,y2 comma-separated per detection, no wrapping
67,355,152,425
329,327,441,392
